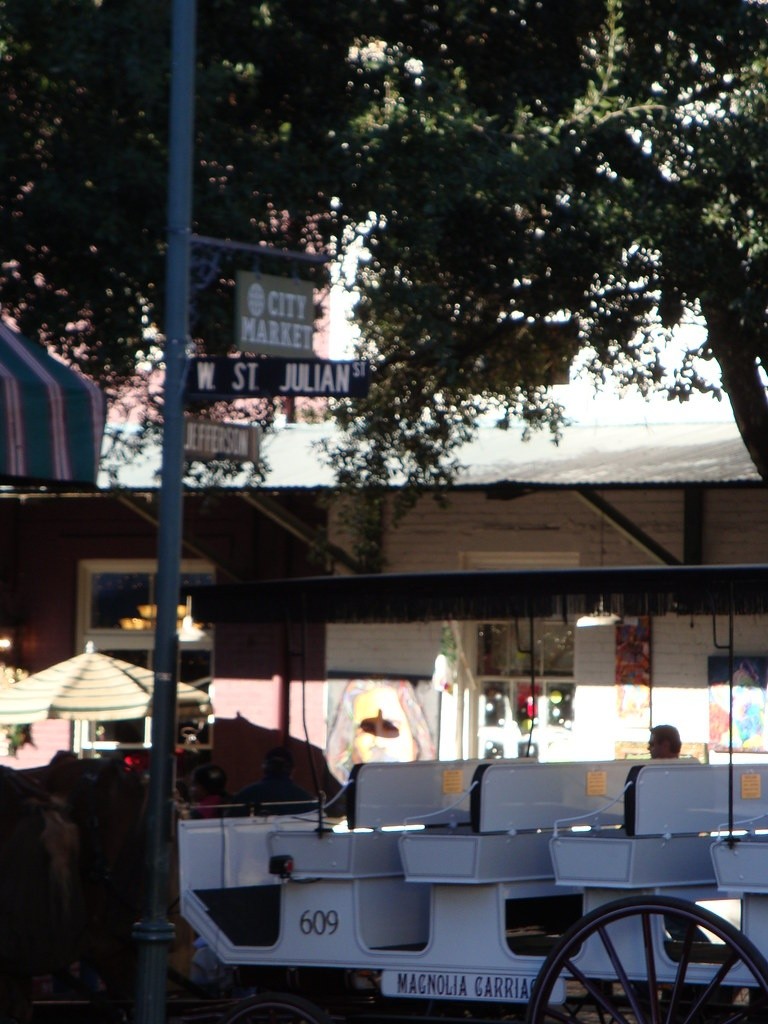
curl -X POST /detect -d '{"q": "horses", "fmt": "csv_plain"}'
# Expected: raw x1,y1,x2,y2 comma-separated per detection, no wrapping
0,757,194,1024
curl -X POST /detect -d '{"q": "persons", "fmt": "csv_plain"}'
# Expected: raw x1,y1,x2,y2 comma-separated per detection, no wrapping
0,722,320,1024
647,725,713,943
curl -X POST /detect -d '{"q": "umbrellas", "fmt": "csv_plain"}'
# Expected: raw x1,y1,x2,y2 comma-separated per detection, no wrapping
0,323,104,486
0,641,213,759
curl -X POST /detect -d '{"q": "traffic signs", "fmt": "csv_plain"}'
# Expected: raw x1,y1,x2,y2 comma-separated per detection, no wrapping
183,415,259,464
183,357,369,401
233,268,316,359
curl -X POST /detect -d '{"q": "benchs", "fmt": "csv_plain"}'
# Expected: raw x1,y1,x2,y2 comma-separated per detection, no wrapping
265,755,768,951
